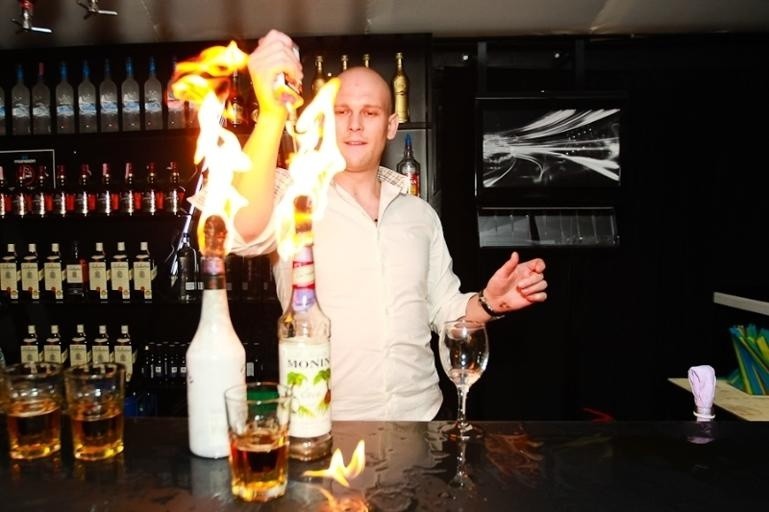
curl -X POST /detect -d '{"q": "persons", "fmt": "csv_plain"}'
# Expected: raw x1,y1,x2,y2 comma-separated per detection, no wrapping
221,27,550,424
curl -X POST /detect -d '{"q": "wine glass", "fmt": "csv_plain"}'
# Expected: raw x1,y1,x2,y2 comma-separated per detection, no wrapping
439,320,489,441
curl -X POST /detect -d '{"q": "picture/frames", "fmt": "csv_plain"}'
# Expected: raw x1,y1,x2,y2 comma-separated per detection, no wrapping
473,92,633,206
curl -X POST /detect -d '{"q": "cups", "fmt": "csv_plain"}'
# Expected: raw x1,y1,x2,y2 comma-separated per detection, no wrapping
225,382,294,501
65,362,127,461
3,361,62,460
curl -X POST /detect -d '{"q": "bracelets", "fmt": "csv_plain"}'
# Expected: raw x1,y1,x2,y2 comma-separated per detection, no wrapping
477,288,508,319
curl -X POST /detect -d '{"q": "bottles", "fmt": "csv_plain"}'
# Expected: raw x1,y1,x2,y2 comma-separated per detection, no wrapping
0,166,11,218
99,64,119,131
32,165,52,216
276,237,331,460
144,58,164,129
363,53,372,67
177,233,198,302
133,242,159,301
110,242,132,300
163,161,186,217
311,53,328,95
391,52,410,123
75,163,97,216
119,162,142,217
227,255,245,299
340,55,350,72
97,162,119,215
246,79,259,131
87,243,110,300
12,164,32,216
30,62,52,134
0,244,20,300
77,61,98,133
226,72,244,129
21,243,43,301
0,88,6,135
272,41,305,111
18,324,43,363
184,256,247,460
144,341,184,385
11,65,30,135
42,325,67,366
55,62,76,134
69,324,91,366
248,259,269,298
113,324,138,383
65,240,86,298
251,343,265,380
121,58,141,130
396,134,421,199
44,244,65,300
91,325,111,368
53,163,75,217
142,161,164,215
166,58,186,128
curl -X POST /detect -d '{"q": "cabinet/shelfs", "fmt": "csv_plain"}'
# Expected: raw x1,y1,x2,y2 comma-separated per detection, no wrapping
0,121,434,393
